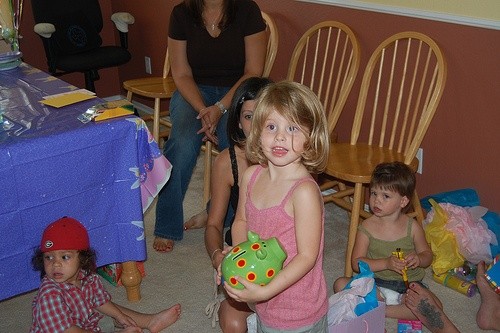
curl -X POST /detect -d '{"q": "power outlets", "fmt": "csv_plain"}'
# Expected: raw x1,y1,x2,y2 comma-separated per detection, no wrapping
144,56,152,75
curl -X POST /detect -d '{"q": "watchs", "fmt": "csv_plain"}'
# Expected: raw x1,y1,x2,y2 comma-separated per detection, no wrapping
214,101,227,115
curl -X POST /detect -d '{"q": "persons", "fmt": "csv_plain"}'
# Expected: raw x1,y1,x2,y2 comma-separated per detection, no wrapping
333,161,444,321
404,261,500,333
152,0,267,253
214,79,328,333
203,77,276,332
30,216,182,333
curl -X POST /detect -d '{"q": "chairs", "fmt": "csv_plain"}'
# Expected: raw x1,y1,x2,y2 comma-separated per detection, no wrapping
159,11,280,211
30,0,142,119
306,30,448,279
287,21,361,222
121,48,179,146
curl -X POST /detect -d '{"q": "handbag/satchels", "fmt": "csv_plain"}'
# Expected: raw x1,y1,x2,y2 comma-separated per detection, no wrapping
328,301,387,333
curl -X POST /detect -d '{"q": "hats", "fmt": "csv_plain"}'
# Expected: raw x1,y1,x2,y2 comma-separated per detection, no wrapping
39,216,89,251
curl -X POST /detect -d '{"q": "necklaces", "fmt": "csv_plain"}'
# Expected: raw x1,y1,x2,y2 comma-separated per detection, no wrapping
202,9,224,30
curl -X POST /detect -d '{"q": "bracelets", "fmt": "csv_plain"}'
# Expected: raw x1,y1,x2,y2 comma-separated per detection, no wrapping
211,248,223,260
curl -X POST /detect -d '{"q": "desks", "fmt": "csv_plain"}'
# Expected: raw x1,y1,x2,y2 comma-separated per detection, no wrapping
0,59,173,304
